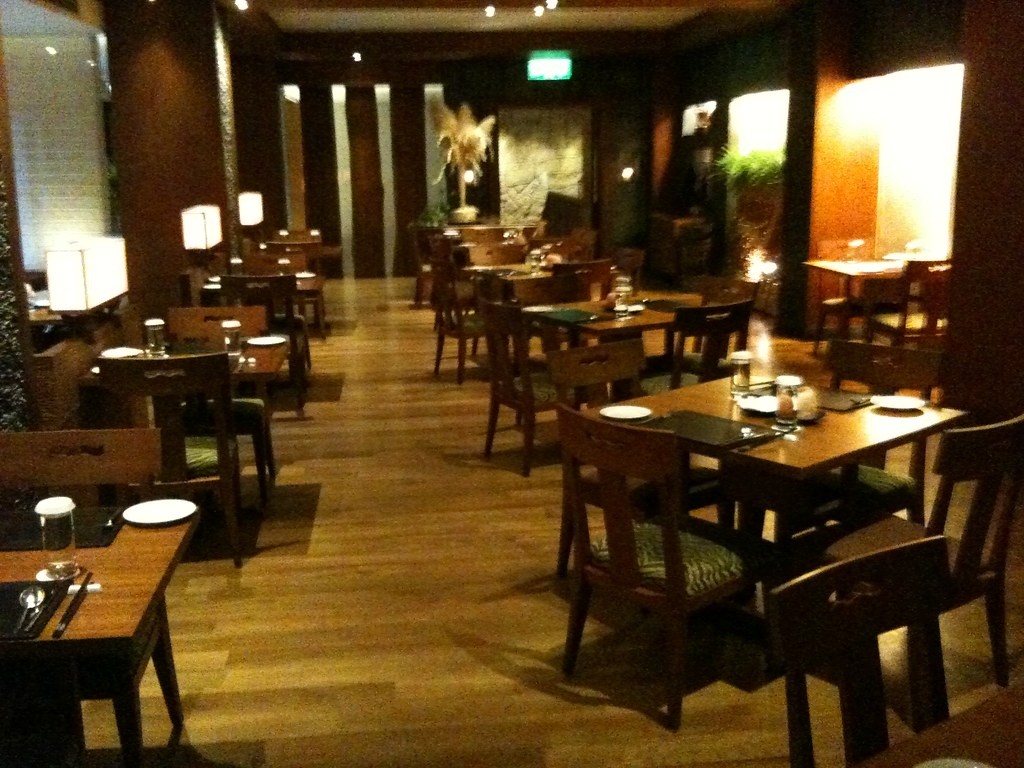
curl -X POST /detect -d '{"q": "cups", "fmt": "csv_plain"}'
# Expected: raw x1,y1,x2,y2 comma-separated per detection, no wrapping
221,321,241,356
530,254,540,275
230,259,243,276
730,358,750,396
145,318,165,353
615,293,629,321
34,497,77,580
277,259,291,276
776,383,798,425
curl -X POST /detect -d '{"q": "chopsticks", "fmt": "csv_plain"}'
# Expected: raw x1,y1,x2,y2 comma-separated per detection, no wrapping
52,571,93,639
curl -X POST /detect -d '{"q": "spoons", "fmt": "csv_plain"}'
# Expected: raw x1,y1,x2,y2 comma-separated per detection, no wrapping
99,508,121,528
12,585,46,634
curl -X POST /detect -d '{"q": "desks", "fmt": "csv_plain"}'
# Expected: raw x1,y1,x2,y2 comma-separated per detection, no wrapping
521,290,730,412
0,509,200,768
577,370,972,584
202,272,327,340
460,264,620,304
79,343,291,426
802,256,950,340
857,676,1024,767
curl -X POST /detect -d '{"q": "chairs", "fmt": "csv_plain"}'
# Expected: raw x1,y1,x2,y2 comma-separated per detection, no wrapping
1,226,1024,768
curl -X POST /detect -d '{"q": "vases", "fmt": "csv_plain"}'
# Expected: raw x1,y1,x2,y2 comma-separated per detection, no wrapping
449,210,477,223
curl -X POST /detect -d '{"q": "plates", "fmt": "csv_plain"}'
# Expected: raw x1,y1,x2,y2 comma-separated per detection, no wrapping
246,336,286,345
599,406,652,420
101,346,141,358
613,305,644,312
123,499,197,524
870,395,925,410
736,394,798,413
296,273,316,279
523,306,551,312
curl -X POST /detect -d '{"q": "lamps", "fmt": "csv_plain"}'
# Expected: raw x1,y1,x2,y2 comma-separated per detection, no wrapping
45,235,130,343
238,192,265,242
181,203,223,272
